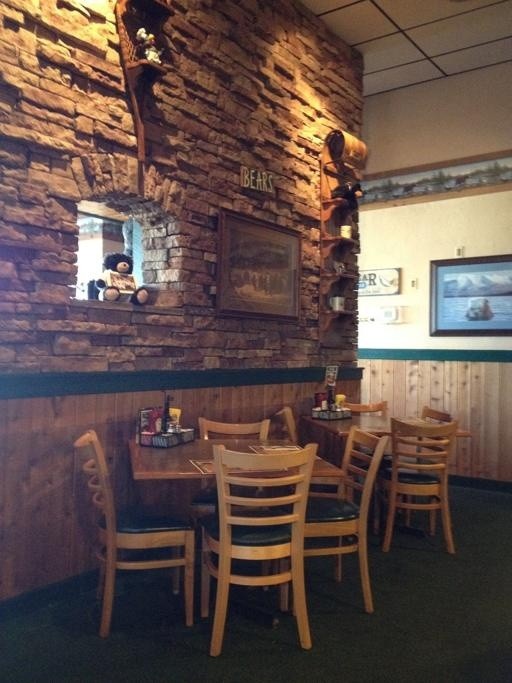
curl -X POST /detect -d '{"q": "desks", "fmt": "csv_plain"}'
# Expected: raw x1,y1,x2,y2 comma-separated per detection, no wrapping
127,435,345,489
300,412,472,439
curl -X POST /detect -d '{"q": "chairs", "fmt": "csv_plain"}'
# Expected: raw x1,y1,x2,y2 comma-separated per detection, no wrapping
200,442,319,656
373,417,455,555
74,429,196,639
384,407,451,537
276,408,353,499
193,416,270,516
263,426,389,613
342,402,392,470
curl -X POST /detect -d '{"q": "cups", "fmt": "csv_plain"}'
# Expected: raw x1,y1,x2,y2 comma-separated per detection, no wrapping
340,226,352,239
329,296,345,311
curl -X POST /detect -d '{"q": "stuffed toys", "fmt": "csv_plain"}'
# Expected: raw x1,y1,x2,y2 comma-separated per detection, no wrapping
96,252,149,304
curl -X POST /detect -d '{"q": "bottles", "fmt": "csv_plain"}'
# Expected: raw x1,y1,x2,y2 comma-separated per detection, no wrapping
166,424,182,434
140,406,170,447
312,390,346,419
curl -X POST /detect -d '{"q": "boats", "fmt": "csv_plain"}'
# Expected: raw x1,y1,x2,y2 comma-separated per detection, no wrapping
466,297,493,320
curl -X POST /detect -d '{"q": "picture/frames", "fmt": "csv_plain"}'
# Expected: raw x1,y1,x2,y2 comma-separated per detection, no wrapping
215,206,302,325
428,254,512,337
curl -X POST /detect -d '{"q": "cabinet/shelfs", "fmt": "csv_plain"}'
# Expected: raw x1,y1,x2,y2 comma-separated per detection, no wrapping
317,130,366,350
114,1,175,162
